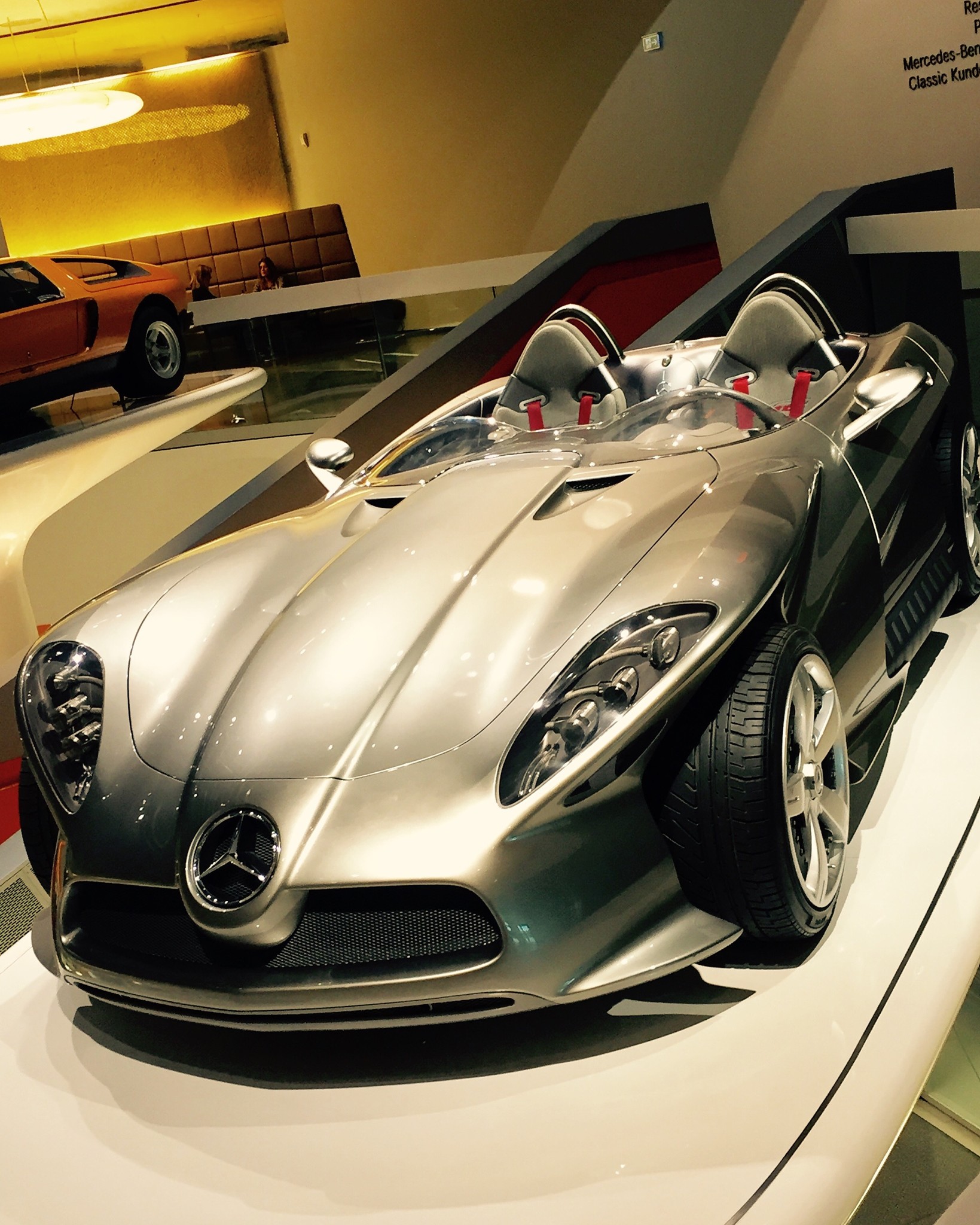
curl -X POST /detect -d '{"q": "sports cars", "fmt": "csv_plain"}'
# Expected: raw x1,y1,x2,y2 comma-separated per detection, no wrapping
10,271,977,1043
1,248,189,401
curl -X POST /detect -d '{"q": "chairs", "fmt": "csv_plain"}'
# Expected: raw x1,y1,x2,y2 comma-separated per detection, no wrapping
693,274,848,436
488,303,631,460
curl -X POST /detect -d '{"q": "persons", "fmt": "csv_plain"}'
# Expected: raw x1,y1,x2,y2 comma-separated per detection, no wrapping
253,257,287,361
189,265,264,365
